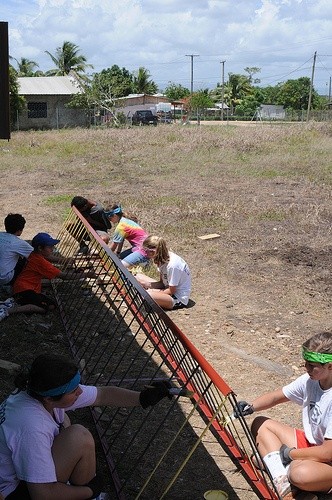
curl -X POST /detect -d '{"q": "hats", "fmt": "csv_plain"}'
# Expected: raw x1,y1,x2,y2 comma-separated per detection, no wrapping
32,232,61,246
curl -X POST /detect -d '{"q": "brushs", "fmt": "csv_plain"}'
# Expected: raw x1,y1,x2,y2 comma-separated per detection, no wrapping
144,384,194,398
218,404,253,429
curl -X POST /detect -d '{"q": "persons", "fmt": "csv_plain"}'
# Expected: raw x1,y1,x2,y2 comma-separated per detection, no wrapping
0,232,99,321
232,332,332,500
102,204,154,270
66,195,112,260
0,213,75,292
134,235,192,310
0,353,176,500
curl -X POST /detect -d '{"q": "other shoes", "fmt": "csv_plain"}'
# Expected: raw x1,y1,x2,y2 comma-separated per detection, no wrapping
279,485,318,500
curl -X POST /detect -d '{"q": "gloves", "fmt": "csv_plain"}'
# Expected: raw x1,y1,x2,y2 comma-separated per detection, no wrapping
139,381,173,409
234,400,254,418
83,477,103,500
280,444,296,469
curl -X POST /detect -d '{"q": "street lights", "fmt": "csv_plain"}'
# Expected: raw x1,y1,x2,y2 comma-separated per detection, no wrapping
186,54,201,126
219,60,228,123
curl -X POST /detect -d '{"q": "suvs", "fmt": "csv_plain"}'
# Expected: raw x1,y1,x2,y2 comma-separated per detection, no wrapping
132,110,158,128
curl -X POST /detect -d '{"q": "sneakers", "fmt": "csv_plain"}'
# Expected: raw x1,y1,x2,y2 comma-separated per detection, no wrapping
0,297,17,322
